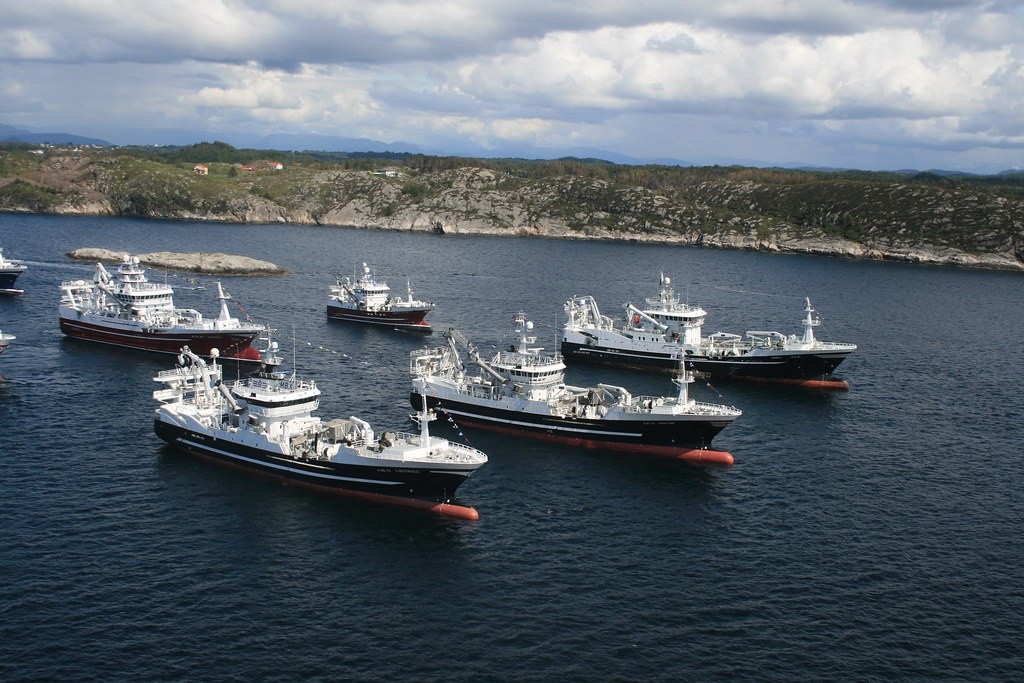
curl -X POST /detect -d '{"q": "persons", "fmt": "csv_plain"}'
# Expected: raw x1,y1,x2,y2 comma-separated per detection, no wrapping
510,345,516,353
634,315,640,323
378,440,387,453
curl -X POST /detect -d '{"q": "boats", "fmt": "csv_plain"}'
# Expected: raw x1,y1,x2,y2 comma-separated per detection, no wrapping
59,254,266,367
152,322,490,521
560,271,858,392
0,247,28,295
409,308,744,467
326,257,436,331
